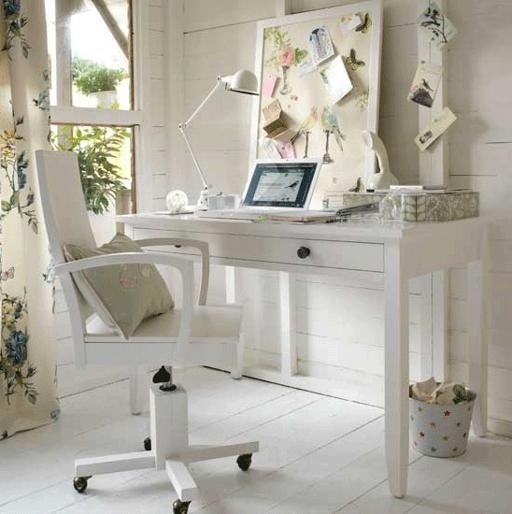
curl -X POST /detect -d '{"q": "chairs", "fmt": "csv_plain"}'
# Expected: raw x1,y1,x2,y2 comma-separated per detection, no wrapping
32,149,259,514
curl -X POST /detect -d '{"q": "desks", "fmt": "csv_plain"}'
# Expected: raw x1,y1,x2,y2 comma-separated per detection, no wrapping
114,204,489,498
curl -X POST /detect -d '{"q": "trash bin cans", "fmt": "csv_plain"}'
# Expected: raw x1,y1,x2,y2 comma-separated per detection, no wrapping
408,382,477,458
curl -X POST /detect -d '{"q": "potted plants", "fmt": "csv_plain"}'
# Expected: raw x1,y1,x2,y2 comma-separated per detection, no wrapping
71,55,129,109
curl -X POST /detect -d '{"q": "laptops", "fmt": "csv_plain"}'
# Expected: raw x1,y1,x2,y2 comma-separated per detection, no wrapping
198,159,322,221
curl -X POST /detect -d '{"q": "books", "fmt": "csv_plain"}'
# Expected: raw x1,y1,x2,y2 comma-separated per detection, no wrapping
271,210,338,223
390,185,448,190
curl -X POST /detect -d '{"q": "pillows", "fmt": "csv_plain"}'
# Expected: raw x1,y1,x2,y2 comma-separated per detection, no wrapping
62,231,176,341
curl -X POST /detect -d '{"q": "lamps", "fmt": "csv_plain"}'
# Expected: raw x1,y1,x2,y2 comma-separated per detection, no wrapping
177,68,260,210
